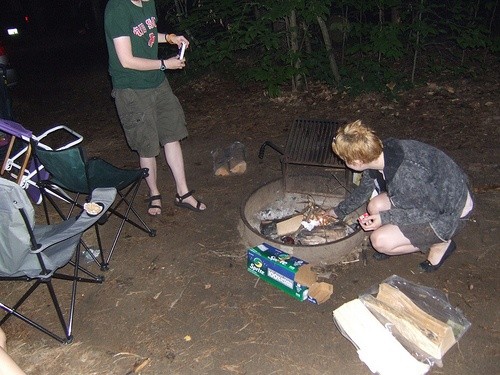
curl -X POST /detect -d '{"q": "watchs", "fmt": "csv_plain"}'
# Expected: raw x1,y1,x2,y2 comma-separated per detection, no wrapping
161,59,165,71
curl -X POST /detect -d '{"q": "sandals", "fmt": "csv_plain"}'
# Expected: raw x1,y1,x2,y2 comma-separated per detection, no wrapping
149,195,162,216
175,190,208,211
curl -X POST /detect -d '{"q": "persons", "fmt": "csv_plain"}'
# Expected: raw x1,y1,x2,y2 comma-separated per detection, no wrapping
323,120,475,272
105,0,207,216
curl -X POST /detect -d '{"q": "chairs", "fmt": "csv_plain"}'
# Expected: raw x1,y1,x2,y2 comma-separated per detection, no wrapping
0,118,157,346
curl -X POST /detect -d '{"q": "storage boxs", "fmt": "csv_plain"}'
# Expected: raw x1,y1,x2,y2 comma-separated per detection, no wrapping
247,243,334,305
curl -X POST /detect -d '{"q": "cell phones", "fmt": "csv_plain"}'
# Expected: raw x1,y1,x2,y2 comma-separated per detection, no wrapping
178,41,185,60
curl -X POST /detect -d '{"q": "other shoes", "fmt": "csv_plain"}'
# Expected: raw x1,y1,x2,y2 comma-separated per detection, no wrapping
420,239,456,272
373,252,390,260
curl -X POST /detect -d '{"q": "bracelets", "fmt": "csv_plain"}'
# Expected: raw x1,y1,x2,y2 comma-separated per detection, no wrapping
165,33,176,45
